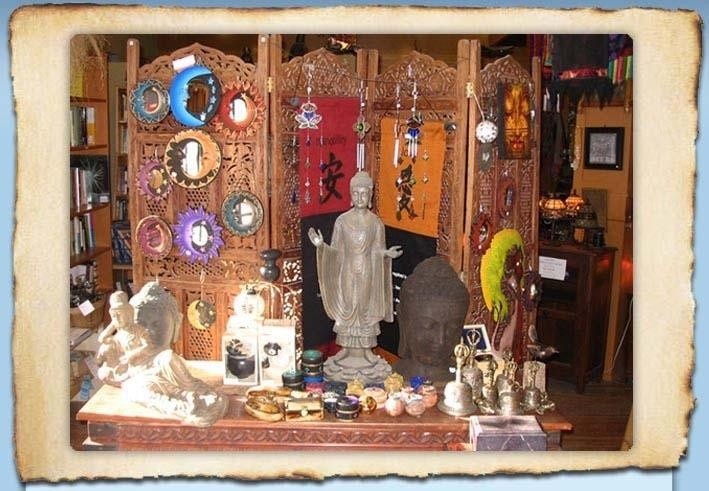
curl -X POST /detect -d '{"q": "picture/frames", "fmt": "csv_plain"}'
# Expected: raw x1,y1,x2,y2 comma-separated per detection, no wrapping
584,127,625,171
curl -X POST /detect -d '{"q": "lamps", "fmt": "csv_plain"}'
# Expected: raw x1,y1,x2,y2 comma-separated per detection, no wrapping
540,189,566,246
571,199,598,250
564,189,584,246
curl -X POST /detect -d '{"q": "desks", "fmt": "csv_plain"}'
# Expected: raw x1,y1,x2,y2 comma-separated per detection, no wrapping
76,359,573,452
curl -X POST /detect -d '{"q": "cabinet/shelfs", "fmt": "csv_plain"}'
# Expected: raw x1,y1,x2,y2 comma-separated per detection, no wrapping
538,251,617,393
70,54,112,292
112,87,127,269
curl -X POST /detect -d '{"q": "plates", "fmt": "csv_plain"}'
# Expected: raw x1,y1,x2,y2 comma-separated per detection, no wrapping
168,64,222,127
134,214,173,261
128,78,170,125
164,128,221,190
186,299,216,331
220,190,265,238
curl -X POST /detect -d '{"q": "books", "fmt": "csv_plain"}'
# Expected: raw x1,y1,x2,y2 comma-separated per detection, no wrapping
69,105,96,149
109,193,131,221
70,214,95,255
72,167,92,213
110,225,132,265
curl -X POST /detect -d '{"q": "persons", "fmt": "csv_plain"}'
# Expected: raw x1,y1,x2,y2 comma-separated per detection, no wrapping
308,172,405,350
389,254,469,387
499,84,533,161
128,278,185,350
94,289,228,426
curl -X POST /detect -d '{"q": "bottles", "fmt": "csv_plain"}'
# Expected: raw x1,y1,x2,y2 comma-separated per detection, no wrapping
383,379,438,418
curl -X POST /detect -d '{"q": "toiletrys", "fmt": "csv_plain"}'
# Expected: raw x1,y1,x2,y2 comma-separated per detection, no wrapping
321,380,386,422
384,372,438,417
